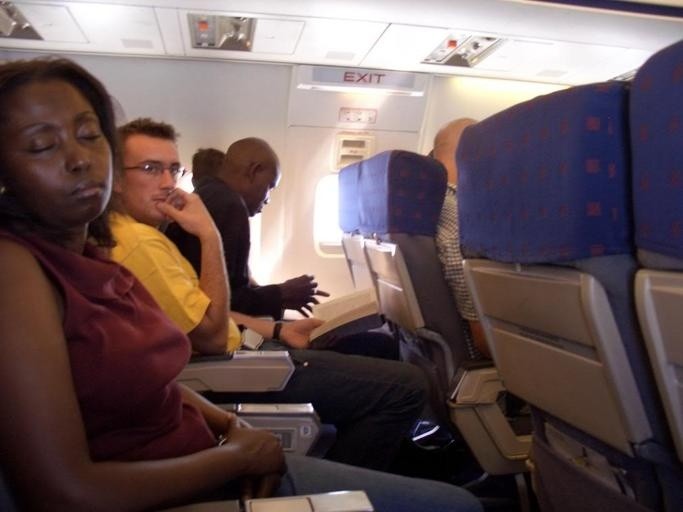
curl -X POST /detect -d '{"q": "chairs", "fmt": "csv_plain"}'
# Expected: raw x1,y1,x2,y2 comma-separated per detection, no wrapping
169,39,682,510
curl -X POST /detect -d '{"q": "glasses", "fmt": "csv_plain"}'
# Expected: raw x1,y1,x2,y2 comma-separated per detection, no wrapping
125,162,186,180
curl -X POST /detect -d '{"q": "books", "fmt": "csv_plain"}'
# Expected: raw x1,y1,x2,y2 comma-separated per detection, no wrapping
308,286,381,342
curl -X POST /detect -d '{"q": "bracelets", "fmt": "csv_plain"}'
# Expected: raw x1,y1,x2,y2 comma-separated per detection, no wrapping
272,319,283,340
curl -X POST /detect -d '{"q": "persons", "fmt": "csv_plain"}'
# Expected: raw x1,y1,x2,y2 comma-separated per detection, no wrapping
191,148,226,189
163,137,330,322
88,118,489,487
0,58,484,512
432,116,493,362
